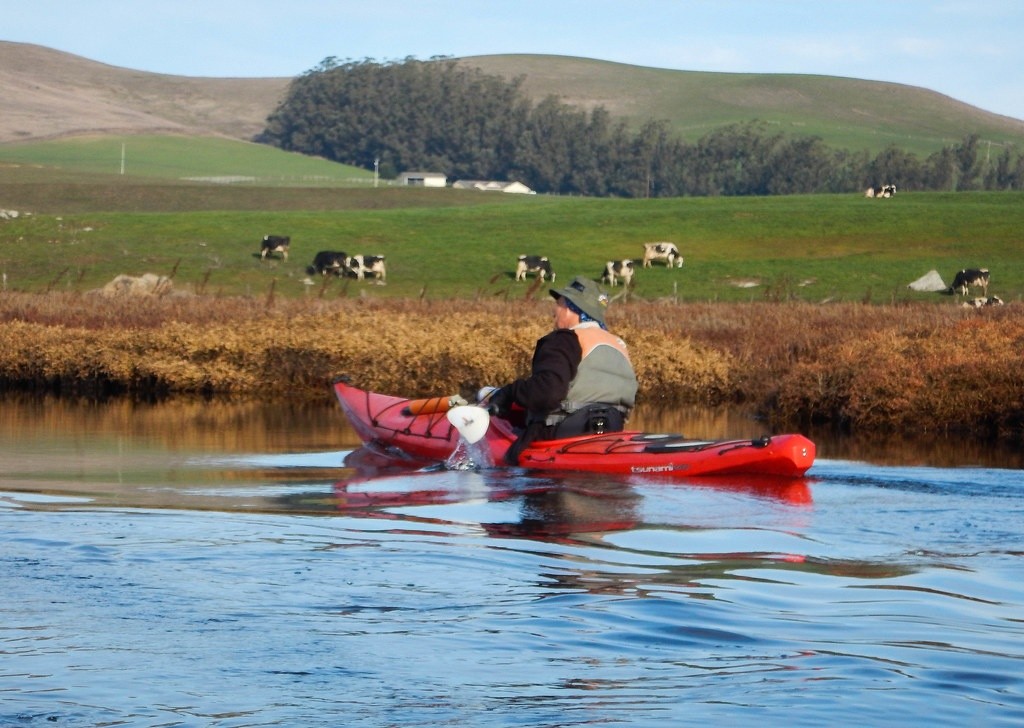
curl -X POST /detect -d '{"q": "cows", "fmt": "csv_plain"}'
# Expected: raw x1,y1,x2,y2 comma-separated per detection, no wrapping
598,259,635,287
515,255,556,283
948,266,991,295
259,234,292,262
964,294,1003,309
863,184,896,199
332,253,387,282
305,250,348,277
644,240,685,268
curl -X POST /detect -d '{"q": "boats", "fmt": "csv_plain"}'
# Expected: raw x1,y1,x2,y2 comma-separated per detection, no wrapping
324,381,817,482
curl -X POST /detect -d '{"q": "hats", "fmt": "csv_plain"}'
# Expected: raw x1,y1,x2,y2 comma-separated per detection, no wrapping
550,276,608,322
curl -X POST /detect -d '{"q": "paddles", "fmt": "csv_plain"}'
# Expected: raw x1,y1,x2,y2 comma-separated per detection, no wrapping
446,403,506,446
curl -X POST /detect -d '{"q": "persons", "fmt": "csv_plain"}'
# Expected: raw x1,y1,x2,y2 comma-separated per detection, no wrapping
487,275,637,442
